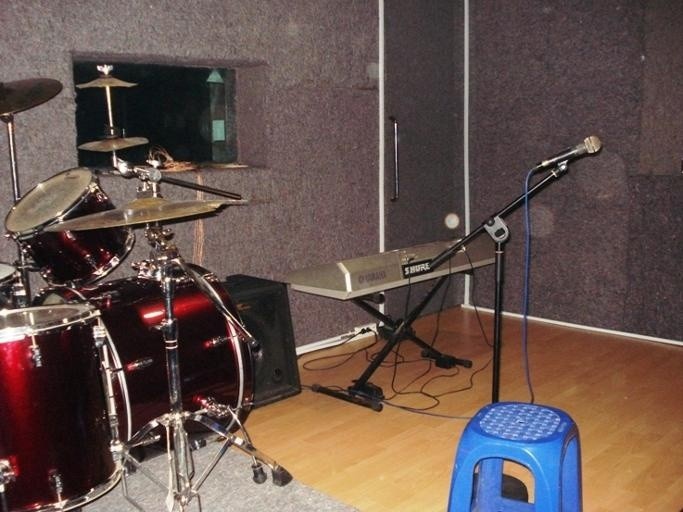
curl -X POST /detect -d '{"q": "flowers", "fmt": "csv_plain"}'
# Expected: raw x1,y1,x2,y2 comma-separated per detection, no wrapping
536,133,603,164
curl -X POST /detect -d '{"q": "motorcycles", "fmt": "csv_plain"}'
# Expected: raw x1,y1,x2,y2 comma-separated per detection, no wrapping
119,243,293,510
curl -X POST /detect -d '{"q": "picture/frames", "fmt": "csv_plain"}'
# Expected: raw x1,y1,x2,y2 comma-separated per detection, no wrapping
292,241,496,411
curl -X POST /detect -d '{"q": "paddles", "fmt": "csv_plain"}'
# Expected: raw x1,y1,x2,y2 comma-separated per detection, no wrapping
82,437,362,512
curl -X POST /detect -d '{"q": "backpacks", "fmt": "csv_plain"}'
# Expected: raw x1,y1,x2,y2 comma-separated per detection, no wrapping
4,167,135,288
0,261,25,307
0,305,122,512
30,264,254,448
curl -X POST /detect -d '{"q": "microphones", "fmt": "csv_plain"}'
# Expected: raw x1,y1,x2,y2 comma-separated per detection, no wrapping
447,401,582,512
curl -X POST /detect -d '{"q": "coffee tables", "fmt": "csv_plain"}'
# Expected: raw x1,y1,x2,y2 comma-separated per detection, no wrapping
77,137,148,154
0,78,62,116
43,197,247,233
75,74,137,87
